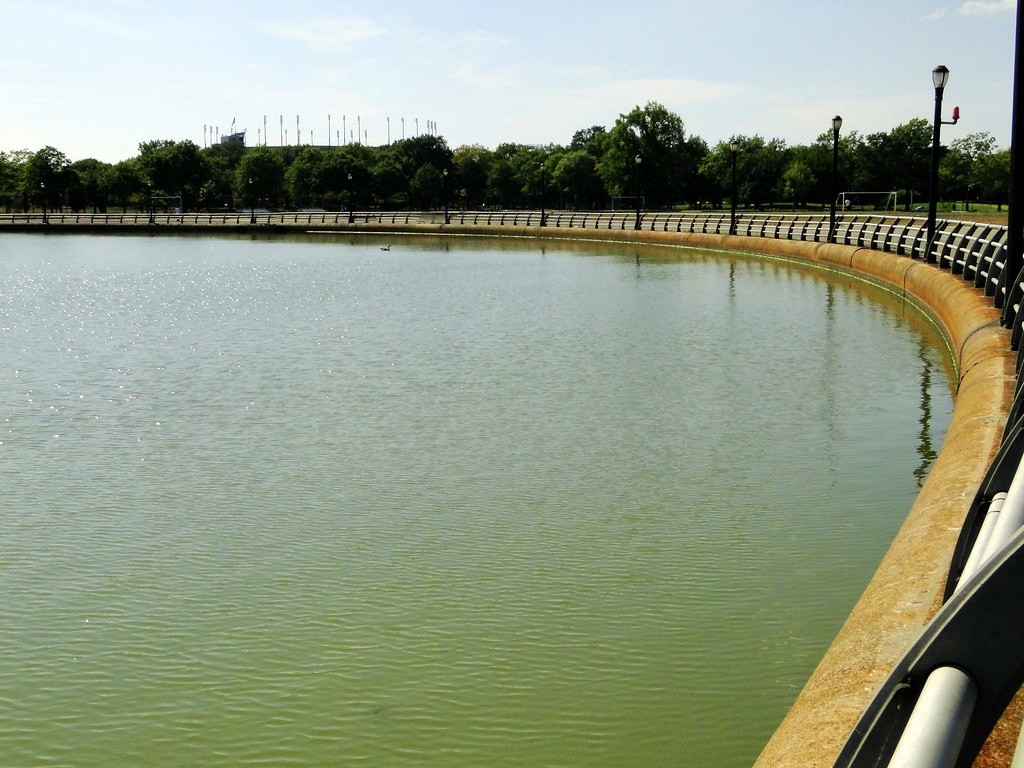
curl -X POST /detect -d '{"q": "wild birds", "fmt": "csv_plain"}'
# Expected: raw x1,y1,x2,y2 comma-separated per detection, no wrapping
380,244,392,252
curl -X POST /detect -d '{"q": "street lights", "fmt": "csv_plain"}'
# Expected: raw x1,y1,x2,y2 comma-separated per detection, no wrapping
830,116,842,233
348,173,354,222
249,178,254,223
927,65,960,246
443,169,448,224
148,181,152,223
635,154,641,230
540,163,546,226
731,141,738,234
41,183,46,222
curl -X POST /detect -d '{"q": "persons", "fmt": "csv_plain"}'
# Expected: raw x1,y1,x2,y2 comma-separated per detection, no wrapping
845,197,851,212
175,207,180,221
